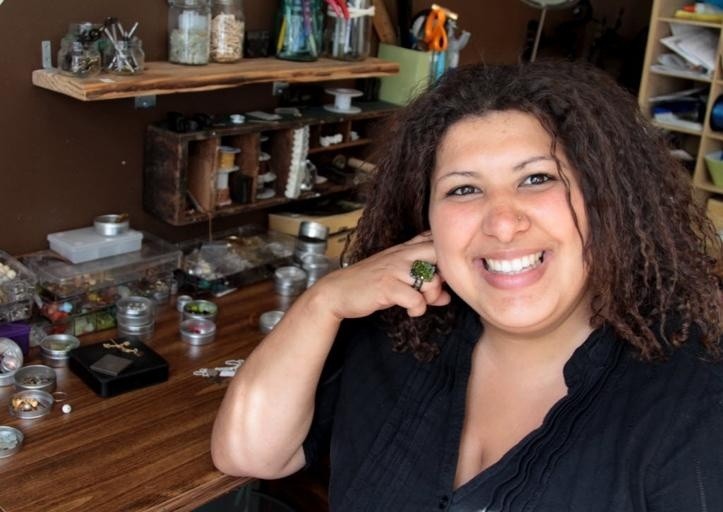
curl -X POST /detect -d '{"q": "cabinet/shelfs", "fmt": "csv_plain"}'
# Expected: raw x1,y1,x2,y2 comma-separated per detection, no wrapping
636,0,723,289
31,54,408,227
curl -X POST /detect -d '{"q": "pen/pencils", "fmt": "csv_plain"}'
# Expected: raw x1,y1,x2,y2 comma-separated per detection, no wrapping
103,21,140,74
277,1,367,59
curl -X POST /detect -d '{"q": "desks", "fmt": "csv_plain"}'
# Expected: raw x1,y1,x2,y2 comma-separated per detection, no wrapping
0,275,299,512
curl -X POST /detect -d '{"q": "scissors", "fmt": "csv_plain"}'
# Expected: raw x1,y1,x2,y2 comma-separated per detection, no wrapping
410,8,449,52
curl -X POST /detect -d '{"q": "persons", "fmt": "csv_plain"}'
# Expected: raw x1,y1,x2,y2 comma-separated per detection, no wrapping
210,53,722,511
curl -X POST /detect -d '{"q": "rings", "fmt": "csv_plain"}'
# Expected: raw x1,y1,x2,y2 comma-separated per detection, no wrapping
409,257,436,288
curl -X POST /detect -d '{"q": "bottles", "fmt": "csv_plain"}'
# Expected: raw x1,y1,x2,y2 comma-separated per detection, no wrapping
212,0,244,63
326,0,374,61
276,0,323,62
167,0,211,67
102,36,145,76
56,39,102,77
